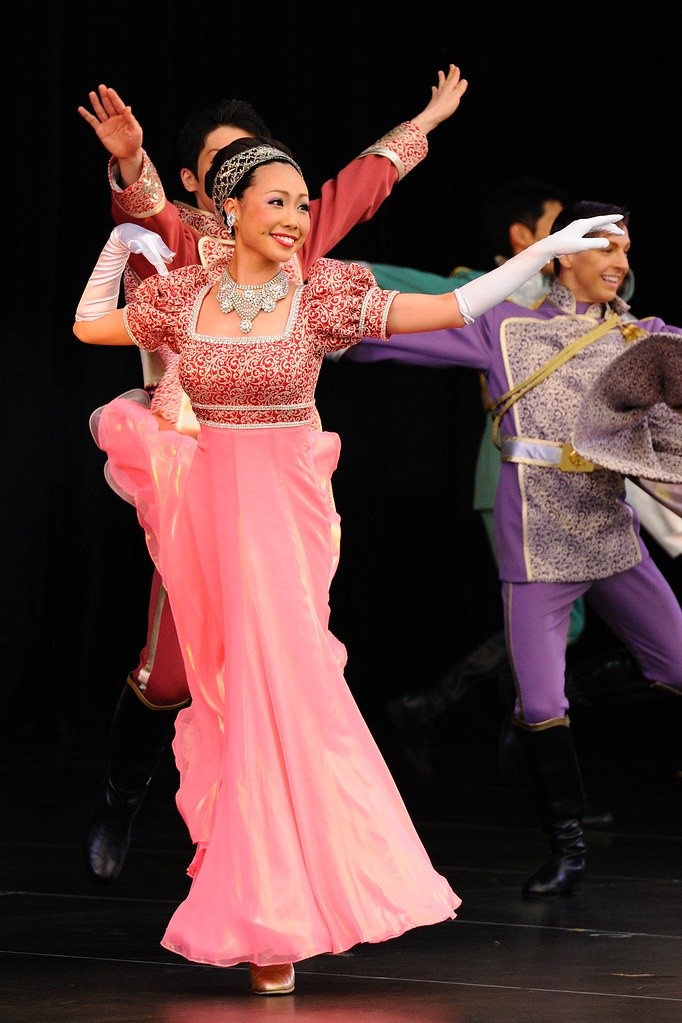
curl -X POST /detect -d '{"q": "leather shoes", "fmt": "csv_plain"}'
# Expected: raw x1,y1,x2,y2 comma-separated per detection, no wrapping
251,964,295,995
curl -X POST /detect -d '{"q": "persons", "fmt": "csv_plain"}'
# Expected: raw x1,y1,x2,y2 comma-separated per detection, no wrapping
79,61,682,905
72,137,627,995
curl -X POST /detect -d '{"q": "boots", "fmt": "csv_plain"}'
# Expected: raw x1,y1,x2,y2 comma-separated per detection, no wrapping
512,714,587,901
92,671,192,880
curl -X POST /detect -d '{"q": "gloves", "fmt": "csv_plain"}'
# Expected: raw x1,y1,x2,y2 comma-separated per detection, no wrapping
75,224,176,323
453,215,624,325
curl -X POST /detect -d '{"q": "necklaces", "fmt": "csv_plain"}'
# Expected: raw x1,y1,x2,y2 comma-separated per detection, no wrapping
217,268,289,335
